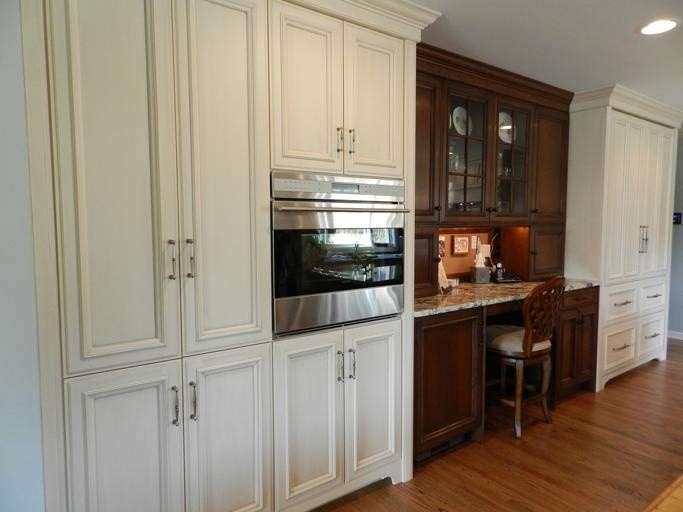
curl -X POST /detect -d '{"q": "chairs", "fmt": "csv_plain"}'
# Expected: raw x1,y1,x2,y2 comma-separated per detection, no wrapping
487,275,565,439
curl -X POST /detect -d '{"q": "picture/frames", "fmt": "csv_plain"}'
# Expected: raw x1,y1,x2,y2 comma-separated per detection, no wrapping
451,234,471,256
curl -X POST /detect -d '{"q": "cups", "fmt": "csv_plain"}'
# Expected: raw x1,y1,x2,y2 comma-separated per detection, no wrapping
447,279,459,288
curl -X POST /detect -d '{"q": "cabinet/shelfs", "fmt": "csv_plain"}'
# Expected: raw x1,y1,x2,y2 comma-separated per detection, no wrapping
499,81,574,278
444,53,535,227
563,82,683,392
558,286,596,400
419,307,480,462
414,42,443,299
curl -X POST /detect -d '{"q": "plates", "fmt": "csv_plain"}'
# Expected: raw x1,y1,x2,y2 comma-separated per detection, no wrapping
452,106,473,136
497,112,517,144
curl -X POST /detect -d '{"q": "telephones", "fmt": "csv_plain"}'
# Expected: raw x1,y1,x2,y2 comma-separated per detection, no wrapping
491,259,522,284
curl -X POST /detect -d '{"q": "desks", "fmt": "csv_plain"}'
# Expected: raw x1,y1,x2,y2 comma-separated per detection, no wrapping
414,274,599,462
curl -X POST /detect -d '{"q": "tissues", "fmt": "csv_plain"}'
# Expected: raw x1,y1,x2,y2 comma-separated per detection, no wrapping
469,253,491,284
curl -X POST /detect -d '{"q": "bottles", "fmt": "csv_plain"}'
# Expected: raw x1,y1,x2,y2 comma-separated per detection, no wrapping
451,201,504,214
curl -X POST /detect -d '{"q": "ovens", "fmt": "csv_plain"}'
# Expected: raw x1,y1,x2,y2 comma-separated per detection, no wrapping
270,168,410,339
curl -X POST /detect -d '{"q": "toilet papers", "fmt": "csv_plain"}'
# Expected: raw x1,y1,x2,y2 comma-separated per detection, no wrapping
476,243,490,254
